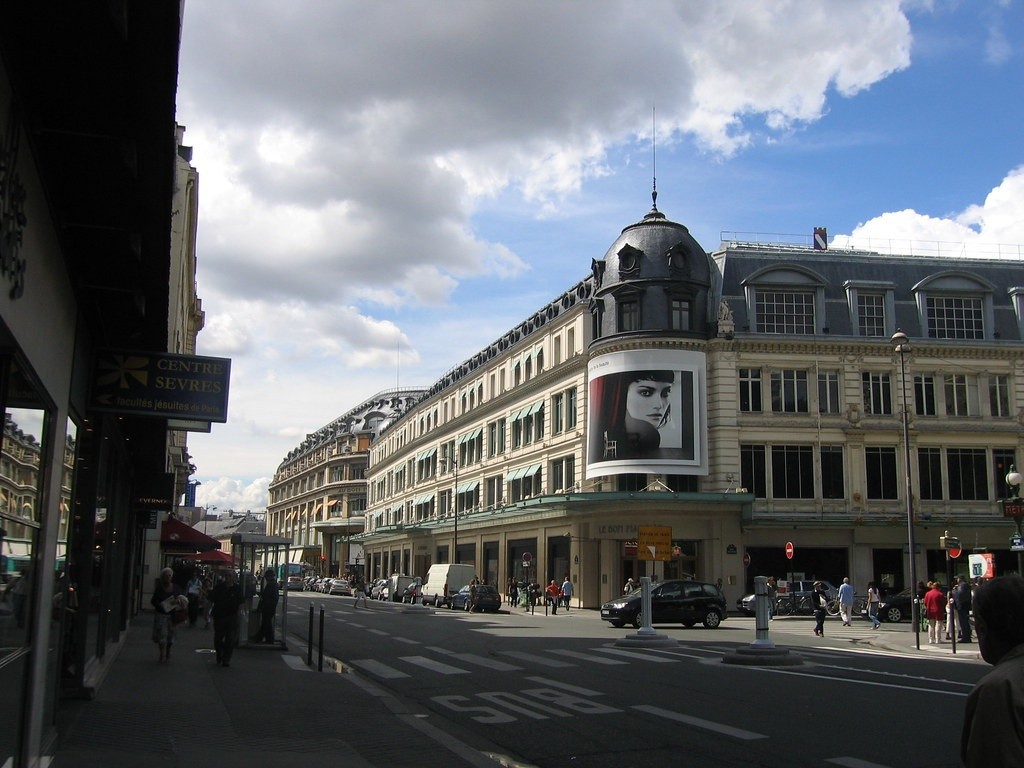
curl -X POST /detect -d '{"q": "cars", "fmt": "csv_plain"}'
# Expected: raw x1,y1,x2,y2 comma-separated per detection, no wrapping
877,584,950,622
451,584,501,611
263,563,420,604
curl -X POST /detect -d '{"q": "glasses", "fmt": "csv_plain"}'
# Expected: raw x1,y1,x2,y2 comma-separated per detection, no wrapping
967,614,987,626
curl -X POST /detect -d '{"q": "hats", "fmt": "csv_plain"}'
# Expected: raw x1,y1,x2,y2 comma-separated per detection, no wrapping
814,582,821,587
954,574,965,578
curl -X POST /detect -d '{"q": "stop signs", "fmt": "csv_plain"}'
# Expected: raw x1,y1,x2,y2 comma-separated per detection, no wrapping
784,542,794,560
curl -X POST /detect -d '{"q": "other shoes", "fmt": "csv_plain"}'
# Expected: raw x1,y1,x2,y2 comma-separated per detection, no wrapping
958,636,962,638
818,632,824,637
958,639,971,643
876,623,880,629
929,639,933,643
843,622,848,626
222,662,230,667
813,628,819,636
936,639,941,643
945,637,951,640
216,659,221,663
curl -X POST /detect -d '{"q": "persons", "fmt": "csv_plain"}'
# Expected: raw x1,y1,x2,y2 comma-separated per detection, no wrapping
469,575,574,614
623,371,676,447
960,573,1024,768
715,578,723,593
251,569,279,643
866,582,881,630
838,577,854,626
169,561,256,667
624,576,641,595
810,581,829,637
918,573,992,643
151,569,190,664
354,576,368,609
379,587,388,601
767,575,778,619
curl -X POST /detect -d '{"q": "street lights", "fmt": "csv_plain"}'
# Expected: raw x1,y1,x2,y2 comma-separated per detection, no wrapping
204,504,218,535
891,332,920,633
1005,465,1024,576
439,456,458,566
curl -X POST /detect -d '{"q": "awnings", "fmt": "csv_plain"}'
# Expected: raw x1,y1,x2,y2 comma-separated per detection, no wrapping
161,518,222,550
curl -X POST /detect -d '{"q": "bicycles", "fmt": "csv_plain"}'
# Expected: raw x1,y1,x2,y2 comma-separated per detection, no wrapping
776,588,867,616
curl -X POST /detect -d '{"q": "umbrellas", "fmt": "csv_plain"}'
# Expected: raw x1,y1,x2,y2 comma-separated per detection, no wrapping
184,551,233,564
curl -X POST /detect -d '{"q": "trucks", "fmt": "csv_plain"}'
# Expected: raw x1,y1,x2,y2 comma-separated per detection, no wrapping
420,563,474,608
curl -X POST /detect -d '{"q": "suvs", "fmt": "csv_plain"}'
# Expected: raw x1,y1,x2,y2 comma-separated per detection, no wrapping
736,580,838,616
600,579,727,628
